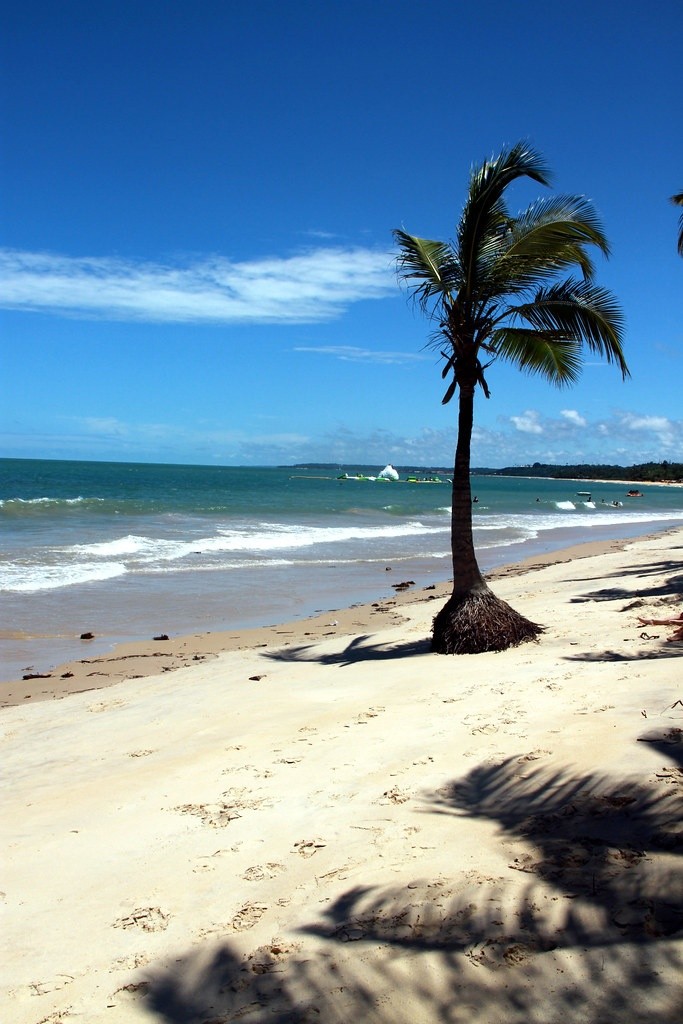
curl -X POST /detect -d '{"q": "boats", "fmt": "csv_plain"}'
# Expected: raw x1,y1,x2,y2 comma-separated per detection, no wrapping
337,472,444,484
626,492,644,497
577,491,591,495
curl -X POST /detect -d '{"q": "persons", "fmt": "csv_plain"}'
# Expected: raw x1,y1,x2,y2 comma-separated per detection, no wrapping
473,497,478,502
601,498,619,507
536,497,541,502
587,497,592,502
